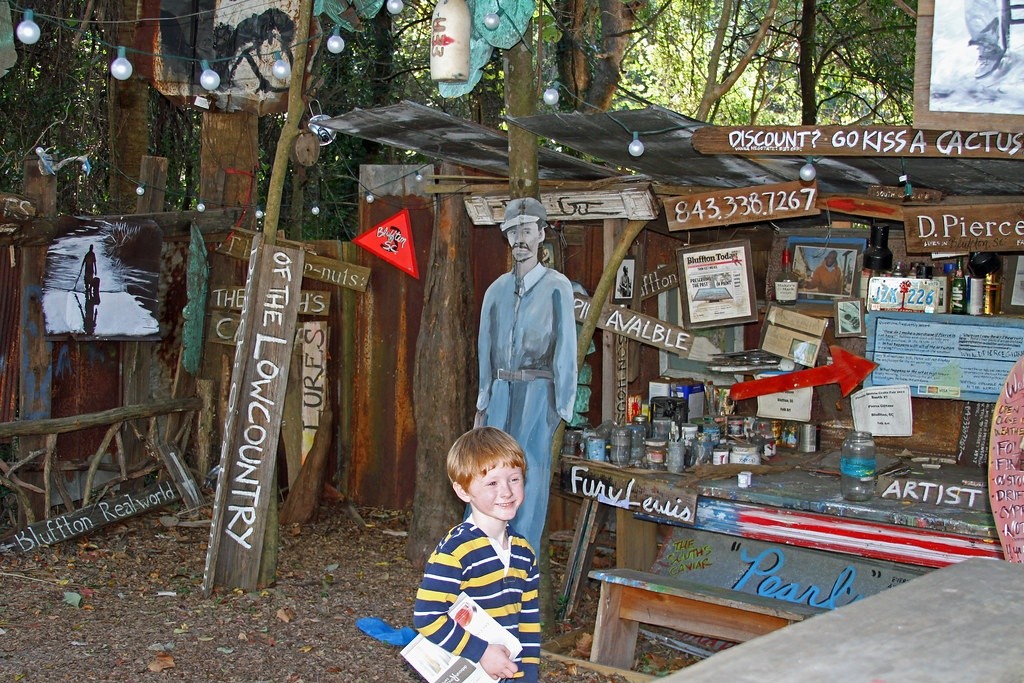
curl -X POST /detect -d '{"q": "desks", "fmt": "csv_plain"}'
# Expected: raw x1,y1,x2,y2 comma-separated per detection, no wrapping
642,557,1024,683
559,442,1005,611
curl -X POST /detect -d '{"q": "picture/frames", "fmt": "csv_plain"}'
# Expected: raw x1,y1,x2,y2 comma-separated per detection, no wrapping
656,264,745,385
785,242,862,298
834,298,867,338
1001,253,1024,316
609,254,636,305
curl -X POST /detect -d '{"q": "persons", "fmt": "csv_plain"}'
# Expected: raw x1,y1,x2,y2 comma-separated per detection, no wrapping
798,250,844,294
412,426,540,683
617,265,632,297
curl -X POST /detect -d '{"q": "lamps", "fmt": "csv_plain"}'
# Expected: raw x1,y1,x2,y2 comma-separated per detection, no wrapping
82,153,423,220
15,0,507,89
544,79,914,199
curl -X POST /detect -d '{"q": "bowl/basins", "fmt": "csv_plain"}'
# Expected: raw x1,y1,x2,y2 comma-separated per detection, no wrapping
970,252,1000,277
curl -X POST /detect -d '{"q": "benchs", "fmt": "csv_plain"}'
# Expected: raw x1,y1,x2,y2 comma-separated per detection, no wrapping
587,568,832,672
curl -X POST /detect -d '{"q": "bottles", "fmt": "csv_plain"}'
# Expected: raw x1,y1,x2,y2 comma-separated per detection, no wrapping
840,432,876,502
984,271,1002,315
751,431,764,453
775,250,798,304
949,257,967,313
632,416,650,439
892,261,905,277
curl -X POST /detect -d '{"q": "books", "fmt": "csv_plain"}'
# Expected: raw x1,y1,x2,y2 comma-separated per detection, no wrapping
400,591,523,683
